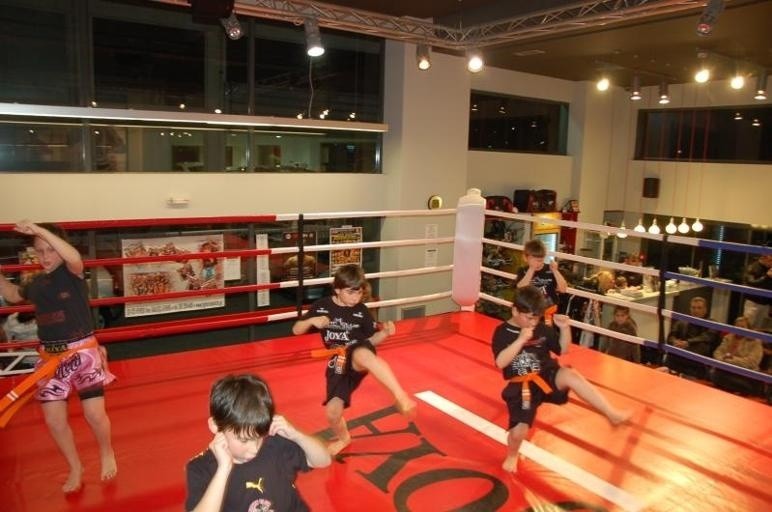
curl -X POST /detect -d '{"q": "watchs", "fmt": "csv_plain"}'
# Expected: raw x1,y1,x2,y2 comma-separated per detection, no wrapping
722,350,733,363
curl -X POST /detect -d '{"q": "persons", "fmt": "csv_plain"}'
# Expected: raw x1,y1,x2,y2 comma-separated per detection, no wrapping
0,219,118,494
512,239,568,329
741,239,772,333
602,306,641,365
491,285,633,474
291,263,417,457
654,296,720,382
710,316,764,397
262,144,282,168
184,372,334,512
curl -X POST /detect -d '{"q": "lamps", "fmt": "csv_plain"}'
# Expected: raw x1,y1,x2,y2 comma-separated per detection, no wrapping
417,42,432,70
648,105,660,235
665,82,677,234
618,73,628,238
220,15,241,40
633,84,646,233
692,81,709,232
465,47,485,73
304,19,326,57
677,82,690,234
599,69,610,239
